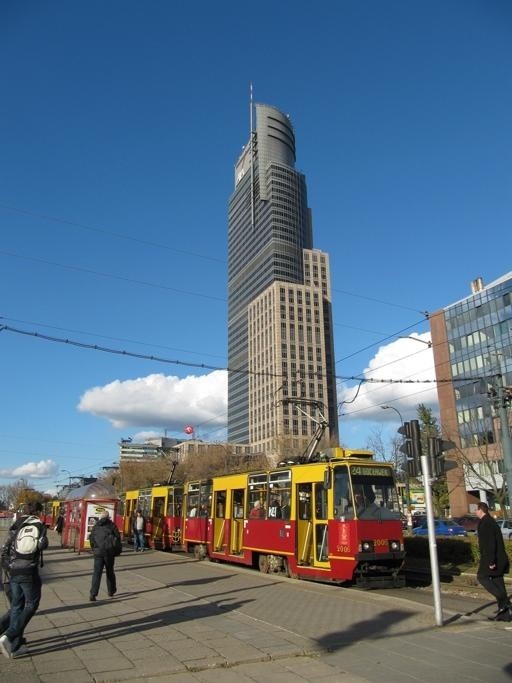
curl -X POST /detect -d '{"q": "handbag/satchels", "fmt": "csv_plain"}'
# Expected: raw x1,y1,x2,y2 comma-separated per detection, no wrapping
104,535,122,556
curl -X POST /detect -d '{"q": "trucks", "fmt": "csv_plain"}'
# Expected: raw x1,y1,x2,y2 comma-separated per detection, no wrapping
413,511,467,538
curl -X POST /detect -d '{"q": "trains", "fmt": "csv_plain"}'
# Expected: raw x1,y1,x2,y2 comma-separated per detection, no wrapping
18,447,406,590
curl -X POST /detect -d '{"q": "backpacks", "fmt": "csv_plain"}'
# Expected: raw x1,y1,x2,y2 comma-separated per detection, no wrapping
14,515,44,555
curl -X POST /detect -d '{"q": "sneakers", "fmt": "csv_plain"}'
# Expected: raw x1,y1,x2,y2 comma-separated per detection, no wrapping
15,648,29,654
0,635,13,658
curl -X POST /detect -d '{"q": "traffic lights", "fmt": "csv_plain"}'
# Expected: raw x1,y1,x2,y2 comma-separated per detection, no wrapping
398,420,422,479
426,437,459,476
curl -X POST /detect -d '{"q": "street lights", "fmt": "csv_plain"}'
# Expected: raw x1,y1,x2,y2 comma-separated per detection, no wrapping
382,404,411,534
62,469,71,485
145,441,168,462
112,461,124,490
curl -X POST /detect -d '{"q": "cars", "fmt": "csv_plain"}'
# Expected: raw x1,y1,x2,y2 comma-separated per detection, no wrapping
392,511,408,530
473,520,512,539
0,510,14,518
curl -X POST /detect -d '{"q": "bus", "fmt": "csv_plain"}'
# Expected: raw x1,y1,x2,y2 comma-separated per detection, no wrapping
375,484,439,527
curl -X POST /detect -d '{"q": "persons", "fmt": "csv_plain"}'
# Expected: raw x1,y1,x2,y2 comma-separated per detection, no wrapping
55,509,64,537
347,489,369,514
130,509,146,553
474,501,511,616
0,500,49,660
154,487,321,520
0,505,28,645
88,510,122,602
